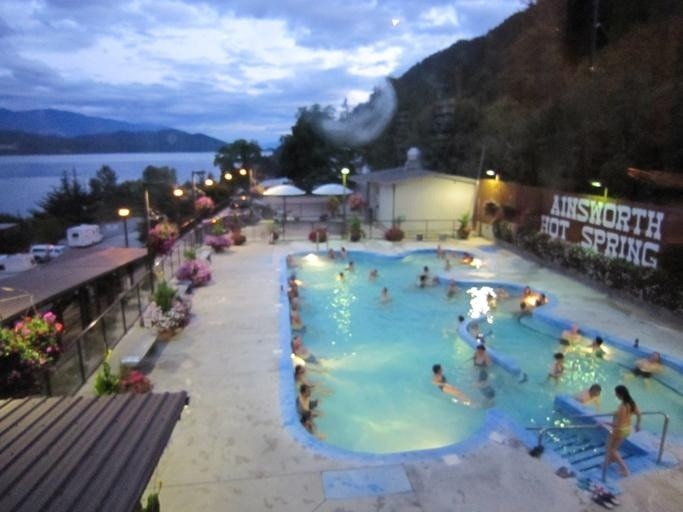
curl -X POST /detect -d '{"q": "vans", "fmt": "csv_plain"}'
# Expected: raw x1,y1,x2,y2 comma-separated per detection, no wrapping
29,245,66,260
66,225,103,248
1,255,36,272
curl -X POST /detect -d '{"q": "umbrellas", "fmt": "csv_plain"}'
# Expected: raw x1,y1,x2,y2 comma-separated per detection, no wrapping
261,183,307,216
311,182,354,219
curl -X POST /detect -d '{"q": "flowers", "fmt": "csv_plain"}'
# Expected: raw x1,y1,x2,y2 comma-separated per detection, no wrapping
141,272,190,330
174,259,210,285
204,234,231,247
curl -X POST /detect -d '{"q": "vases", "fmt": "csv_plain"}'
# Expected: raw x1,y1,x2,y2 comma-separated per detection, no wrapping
158,325,176,339
213,245,225,253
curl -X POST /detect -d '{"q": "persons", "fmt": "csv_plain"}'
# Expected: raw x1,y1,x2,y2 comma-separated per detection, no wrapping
379,287,392,304
601,384,642,477
623,351,664,395
544,351,566,387
286,253,303,269
572,383,601,413
286,274,333,441
328,246,378,282
415,243,547,408
577,335,606,363
552,320,582,355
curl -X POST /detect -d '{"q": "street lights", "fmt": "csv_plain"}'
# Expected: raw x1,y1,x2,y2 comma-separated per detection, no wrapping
172,167,247,224
342,167,350,237
118,208,130,248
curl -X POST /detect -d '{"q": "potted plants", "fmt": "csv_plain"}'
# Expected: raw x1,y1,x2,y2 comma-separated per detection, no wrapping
348,214,365,241
457,211,471,238
